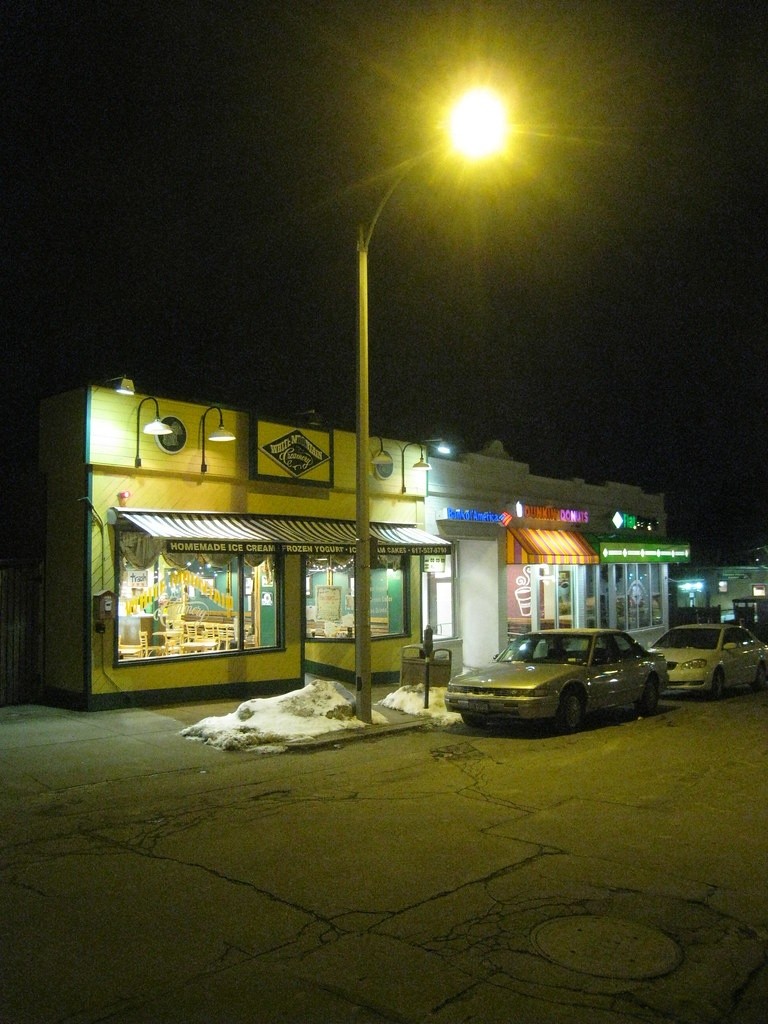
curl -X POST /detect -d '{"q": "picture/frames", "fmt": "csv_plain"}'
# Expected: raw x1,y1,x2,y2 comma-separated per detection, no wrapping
201,576,216,598
306,576,313,598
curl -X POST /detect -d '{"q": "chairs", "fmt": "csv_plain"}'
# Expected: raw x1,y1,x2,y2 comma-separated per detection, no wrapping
139,621,234,658
547,649,566,660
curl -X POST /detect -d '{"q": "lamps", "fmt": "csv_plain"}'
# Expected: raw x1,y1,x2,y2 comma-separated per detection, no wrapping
99,374,135,396
368,433,392,464
427,438,451,453
201,405,235,471
402,442,431,492
134,396,173,468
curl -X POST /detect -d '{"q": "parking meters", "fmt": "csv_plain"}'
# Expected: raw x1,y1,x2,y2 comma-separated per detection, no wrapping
422,623,433,711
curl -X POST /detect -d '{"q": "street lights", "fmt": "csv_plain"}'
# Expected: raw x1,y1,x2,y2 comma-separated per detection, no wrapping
352,83,510,724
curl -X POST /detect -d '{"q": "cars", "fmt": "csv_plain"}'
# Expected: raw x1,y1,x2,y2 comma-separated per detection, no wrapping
443,627,670,735
648,624,768,700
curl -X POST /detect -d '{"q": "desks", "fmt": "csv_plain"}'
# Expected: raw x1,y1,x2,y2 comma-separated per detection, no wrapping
182,640,217,654
